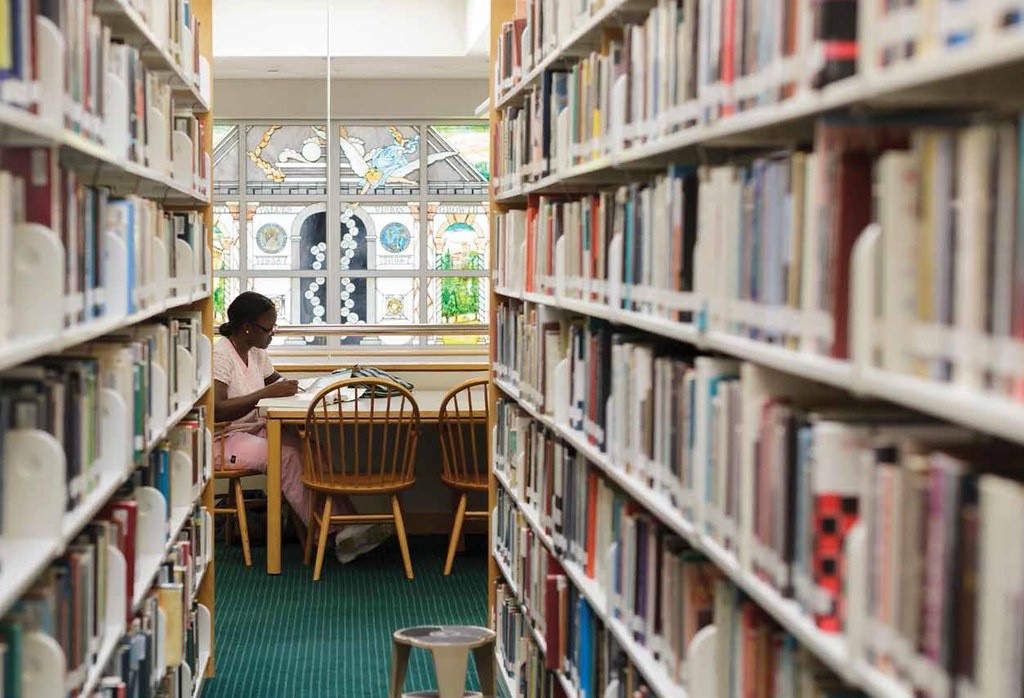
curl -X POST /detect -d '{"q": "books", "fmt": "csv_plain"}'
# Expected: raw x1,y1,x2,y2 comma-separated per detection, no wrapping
491,0,1024,698
254,373,351,408
1,0,211,698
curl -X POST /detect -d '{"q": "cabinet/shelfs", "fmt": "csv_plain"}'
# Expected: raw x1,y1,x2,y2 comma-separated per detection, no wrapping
0,0,217,698
488,0,1024,698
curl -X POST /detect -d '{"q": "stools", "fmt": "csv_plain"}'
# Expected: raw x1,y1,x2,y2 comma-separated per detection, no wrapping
389,625,496,698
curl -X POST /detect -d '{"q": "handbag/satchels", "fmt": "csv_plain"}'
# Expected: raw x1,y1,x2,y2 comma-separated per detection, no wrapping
331,364,415,399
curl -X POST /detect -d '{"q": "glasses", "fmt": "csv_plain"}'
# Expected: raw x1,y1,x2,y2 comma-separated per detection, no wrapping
254,323,279,336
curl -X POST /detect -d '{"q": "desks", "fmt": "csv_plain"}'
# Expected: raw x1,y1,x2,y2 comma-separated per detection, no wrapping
254,371,489,575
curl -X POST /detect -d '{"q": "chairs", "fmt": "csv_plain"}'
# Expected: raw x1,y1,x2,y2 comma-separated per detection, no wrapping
214,422,252,566
438,376,489,575
297,377,420,580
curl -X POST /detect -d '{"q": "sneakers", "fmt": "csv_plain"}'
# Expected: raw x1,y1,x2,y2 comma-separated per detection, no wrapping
336,545,377,564
334,523,397,554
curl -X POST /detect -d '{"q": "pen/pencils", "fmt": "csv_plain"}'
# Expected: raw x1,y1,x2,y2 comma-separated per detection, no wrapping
284,375,307,392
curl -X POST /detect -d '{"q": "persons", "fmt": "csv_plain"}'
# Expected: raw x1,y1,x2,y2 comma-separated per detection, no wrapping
211,290,395,564
